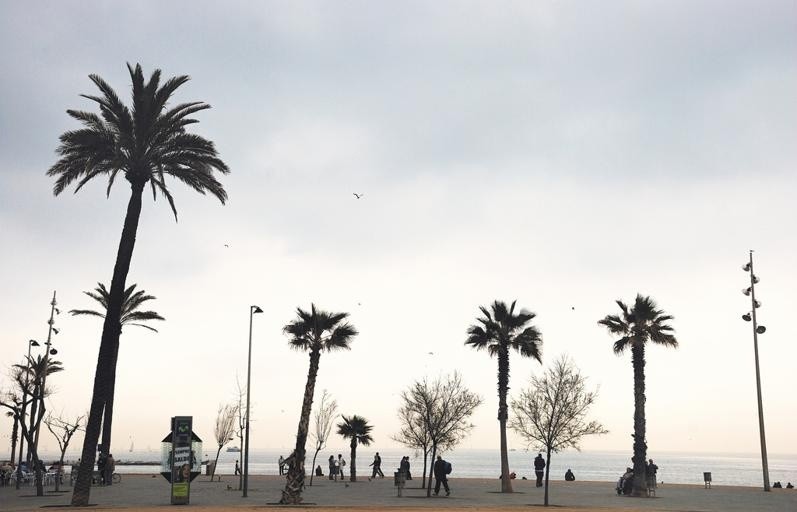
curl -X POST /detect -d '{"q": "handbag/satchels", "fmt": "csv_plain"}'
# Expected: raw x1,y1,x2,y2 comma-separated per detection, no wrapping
342,459,346,466
444,462,451,474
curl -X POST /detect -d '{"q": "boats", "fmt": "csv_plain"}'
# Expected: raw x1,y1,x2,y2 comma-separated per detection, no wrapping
225,445,241,453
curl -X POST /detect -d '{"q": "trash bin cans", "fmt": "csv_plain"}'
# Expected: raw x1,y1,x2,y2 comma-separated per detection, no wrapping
206,462,214,475
394,467,405,488
704,472,711,482
647,474,657,490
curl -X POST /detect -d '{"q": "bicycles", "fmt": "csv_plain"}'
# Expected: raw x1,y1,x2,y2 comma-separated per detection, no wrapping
110,471,122,484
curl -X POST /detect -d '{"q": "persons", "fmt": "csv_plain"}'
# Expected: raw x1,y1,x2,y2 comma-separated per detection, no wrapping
1,452,115,489
432,455,451,496
533,453,545,488
178,462,190,484
616,468,634,490
233,459,240,476
564,468,574,481
314,448,414,482
278,455,286,476
648,458,658,487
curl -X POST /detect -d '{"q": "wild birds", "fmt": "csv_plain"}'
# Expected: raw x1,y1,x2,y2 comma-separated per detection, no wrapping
353,193,364,199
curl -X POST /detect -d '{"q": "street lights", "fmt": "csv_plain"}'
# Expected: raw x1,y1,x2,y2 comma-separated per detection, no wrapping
14,338,40,491
27,289,60,472
740,247,772,494
240,305,265,498
